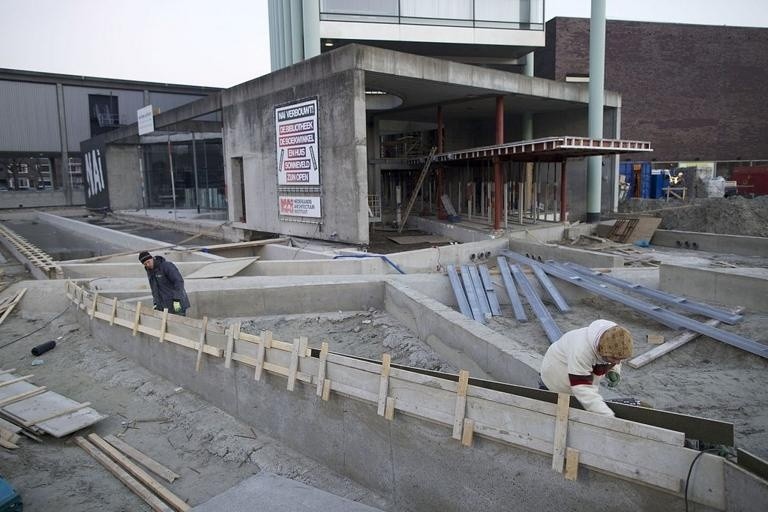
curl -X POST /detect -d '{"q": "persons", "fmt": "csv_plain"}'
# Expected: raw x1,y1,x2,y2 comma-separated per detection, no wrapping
137,250,192,317
536,314,634,418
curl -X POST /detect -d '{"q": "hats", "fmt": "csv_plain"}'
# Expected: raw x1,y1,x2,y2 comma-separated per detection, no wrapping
599,325,634,359
139,251,152,264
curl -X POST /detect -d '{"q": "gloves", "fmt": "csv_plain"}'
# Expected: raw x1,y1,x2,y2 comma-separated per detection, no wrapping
604,371,620,387
172,298,182,312
152,303,157,310
